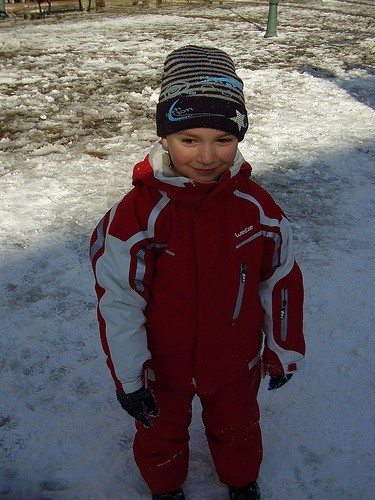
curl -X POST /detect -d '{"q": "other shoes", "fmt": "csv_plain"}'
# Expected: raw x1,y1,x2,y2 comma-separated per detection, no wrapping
228,481,260,500
152,488,185,500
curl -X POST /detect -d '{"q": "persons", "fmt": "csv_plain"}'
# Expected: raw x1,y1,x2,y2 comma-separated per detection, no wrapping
88,45,306,500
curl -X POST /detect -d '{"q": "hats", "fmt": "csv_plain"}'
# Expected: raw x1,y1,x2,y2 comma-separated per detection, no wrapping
155,44,248,145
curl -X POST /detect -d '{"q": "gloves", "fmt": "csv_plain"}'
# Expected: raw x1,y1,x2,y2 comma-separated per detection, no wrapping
116,385,160,429
263,373,294,391
